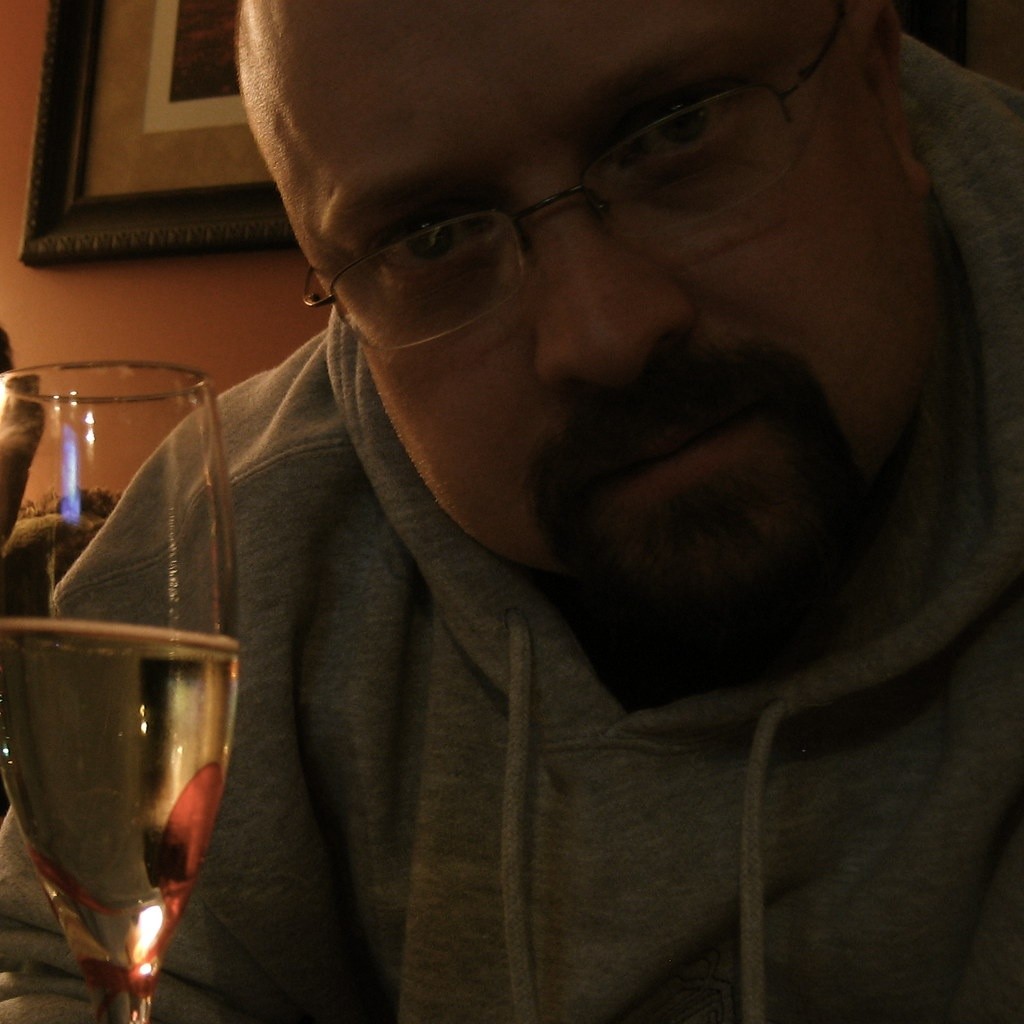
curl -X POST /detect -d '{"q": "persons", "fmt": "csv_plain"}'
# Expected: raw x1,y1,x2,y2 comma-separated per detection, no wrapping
0,0,1024,1023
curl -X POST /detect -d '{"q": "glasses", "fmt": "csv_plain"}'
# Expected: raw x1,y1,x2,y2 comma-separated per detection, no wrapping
302,5,847,351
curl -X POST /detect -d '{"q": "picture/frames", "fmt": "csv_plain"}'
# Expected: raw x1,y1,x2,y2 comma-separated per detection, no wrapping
16,0,301,266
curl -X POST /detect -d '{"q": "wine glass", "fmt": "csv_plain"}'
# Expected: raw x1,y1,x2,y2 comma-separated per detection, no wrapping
0,361,241,1023
